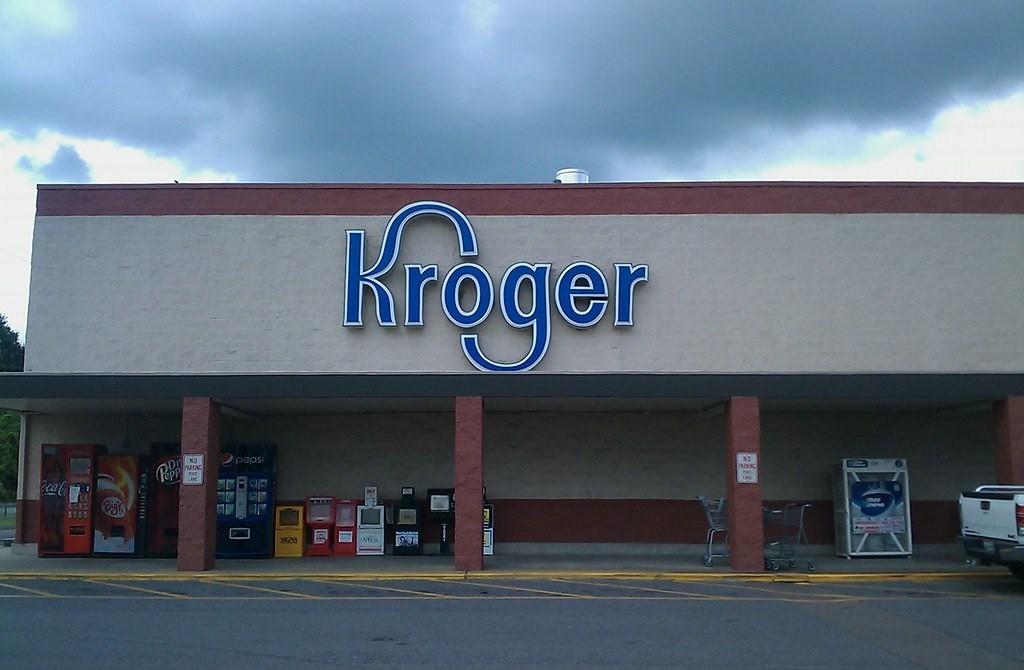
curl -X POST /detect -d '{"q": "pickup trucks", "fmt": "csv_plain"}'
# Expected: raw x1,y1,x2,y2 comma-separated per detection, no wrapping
959,485,1024,582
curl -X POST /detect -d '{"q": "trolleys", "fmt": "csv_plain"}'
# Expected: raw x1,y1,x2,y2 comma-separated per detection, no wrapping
695,495,816,572
